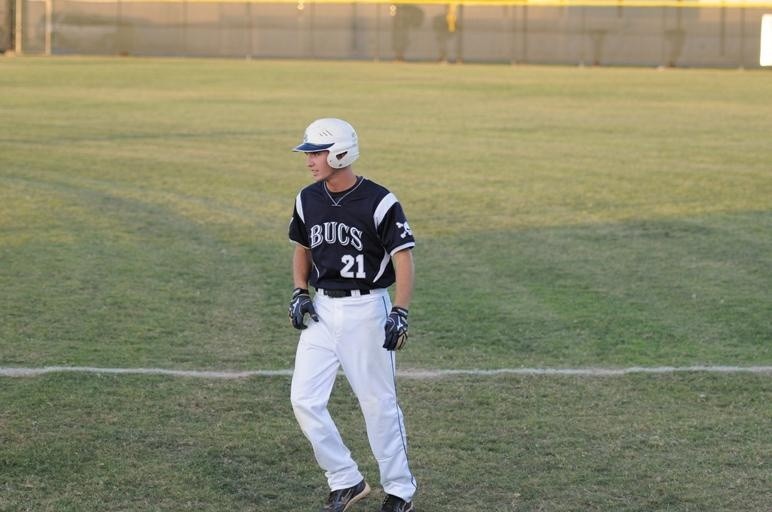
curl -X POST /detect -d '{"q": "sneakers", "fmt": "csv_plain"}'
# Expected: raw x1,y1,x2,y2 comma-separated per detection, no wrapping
378,494,414,512
323,480,371,512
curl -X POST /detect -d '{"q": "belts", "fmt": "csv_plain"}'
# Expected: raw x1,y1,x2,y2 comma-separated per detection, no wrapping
315,287,370,297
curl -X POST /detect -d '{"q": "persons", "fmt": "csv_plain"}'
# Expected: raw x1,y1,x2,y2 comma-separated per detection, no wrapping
287,118,418,512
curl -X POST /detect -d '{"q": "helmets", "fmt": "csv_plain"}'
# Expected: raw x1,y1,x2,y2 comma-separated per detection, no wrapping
292,118,361,169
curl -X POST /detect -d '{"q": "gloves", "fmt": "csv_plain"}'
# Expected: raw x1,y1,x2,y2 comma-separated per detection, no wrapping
382,307,408,351
288,288,319,330
